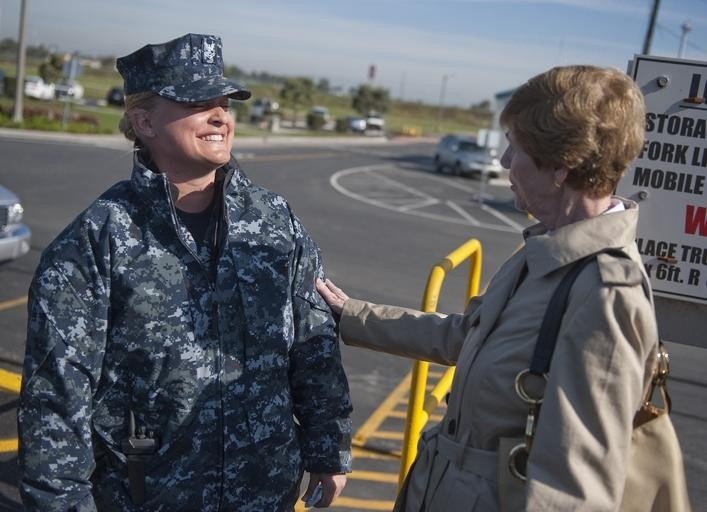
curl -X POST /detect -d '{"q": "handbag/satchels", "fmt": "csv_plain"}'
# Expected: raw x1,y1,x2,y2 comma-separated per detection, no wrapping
496,403,693,512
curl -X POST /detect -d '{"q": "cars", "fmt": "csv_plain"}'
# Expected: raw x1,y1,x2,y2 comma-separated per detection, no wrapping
0,182,32,265
24,74,123,112
434,133,501,180
249,98,384,133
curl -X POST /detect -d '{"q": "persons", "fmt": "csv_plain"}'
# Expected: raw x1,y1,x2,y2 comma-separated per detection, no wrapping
316,63,693,512
15,33,353,512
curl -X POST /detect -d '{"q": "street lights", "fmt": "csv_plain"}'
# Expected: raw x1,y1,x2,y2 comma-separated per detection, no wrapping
677,19,692,56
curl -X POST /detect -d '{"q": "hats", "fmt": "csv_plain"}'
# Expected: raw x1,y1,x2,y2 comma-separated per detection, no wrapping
116,32,252,104
